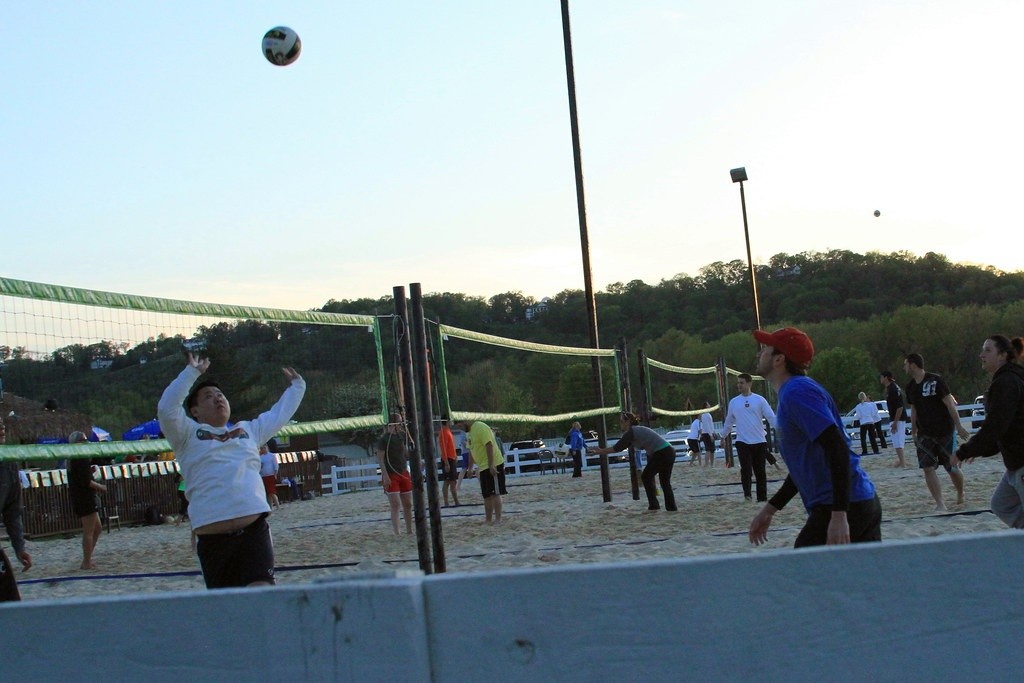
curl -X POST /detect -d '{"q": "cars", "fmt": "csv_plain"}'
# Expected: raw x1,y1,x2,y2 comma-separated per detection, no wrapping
664,430,690,453
584,438,628,466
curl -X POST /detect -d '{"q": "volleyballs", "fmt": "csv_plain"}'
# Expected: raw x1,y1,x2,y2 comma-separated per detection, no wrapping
261,26,301,66
874,210,880,218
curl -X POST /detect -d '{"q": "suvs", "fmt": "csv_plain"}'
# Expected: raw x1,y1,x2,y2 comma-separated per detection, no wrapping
972,396,987,429
507,439,546,474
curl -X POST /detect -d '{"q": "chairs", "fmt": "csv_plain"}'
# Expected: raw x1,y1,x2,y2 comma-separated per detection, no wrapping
538,444,571,475
98,498,119,534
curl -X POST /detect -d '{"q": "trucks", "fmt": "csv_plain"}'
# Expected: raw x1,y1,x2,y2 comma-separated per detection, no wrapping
846,400,912,436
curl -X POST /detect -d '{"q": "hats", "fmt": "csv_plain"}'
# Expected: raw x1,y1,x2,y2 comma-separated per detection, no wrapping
754,327,813,369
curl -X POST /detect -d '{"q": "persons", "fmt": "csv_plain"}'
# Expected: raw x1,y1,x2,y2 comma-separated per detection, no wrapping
880,370,908,468
950,335,1024,531
67,432,280,572
903,353,969,513
437,414,508,525
377,412,413,536
866,395,888,449
749,328,882,549
719,373,779,503
157,352,306,590
0,416,31,601
593,412,678,515
855,392,881,455
687,402,784,472
569,422,589,478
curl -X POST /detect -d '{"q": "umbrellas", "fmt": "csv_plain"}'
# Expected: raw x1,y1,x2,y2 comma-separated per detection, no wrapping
87,417,166,442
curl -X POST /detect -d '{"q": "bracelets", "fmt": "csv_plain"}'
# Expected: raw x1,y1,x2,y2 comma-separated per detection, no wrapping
95,483,101,488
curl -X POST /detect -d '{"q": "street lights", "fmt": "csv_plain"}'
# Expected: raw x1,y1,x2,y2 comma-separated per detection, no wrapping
729,167,772,406
